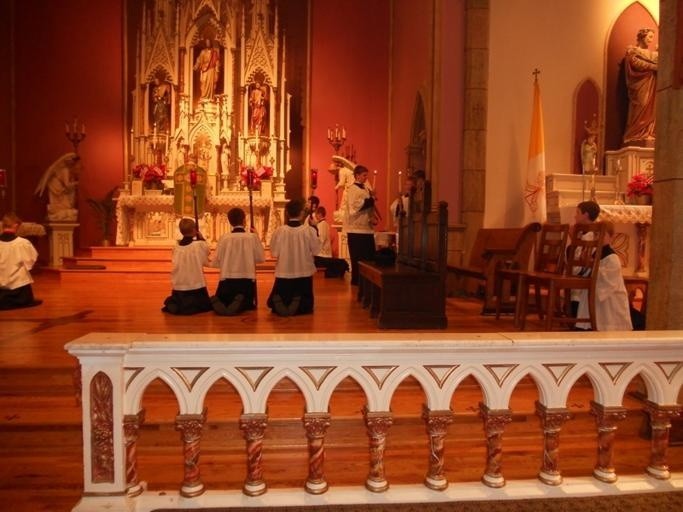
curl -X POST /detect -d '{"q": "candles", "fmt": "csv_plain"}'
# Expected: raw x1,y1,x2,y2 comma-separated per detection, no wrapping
254,126,259,151
324,123,347,142
269,135,274,162
153,126,157,148
163,135,169,160
129,133,134,158
237,132,240,162
371,173,376,193
62,114,84,136
397,172,401,197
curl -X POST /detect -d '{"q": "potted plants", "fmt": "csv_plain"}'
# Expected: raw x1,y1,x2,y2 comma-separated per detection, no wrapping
81,185,125,244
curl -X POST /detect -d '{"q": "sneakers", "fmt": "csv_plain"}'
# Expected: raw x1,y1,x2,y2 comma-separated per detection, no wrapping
273,294,289,317
210,295,226,316
225,295,243,316
287,296,300,316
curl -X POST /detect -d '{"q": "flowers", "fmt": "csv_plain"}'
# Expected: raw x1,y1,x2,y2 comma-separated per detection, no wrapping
133,161,169,188
237,165,273,191
621,172,653,204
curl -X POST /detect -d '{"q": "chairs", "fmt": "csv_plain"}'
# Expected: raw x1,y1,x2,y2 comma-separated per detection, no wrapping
494,221,572,329
516,218,608,333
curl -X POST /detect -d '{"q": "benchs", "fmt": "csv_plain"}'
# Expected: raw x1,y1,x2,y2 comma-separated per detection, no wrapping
353,256,447,332
445,220,540,310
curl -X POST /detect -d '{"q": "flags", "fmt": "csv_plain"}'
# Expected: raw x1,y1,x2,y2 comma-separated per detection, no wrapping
520,77,548,272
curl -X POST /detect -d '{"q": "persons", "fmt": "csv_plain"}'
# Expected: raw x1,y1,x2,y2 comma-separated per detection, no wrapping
266,198,319,316
574,221,634,333
327,163,355,223
303,196,320,227
161,218,211,315
410,170,427,212
343,165,378,284
0,213,41,309
150,79,169,133
247,79,267,135
623,28,657,148
193,37,221,100
389,177,419,256
209,207,262,315
580,136,598,175
46,153,81,222
313,207,330,256
562,201,599,328
